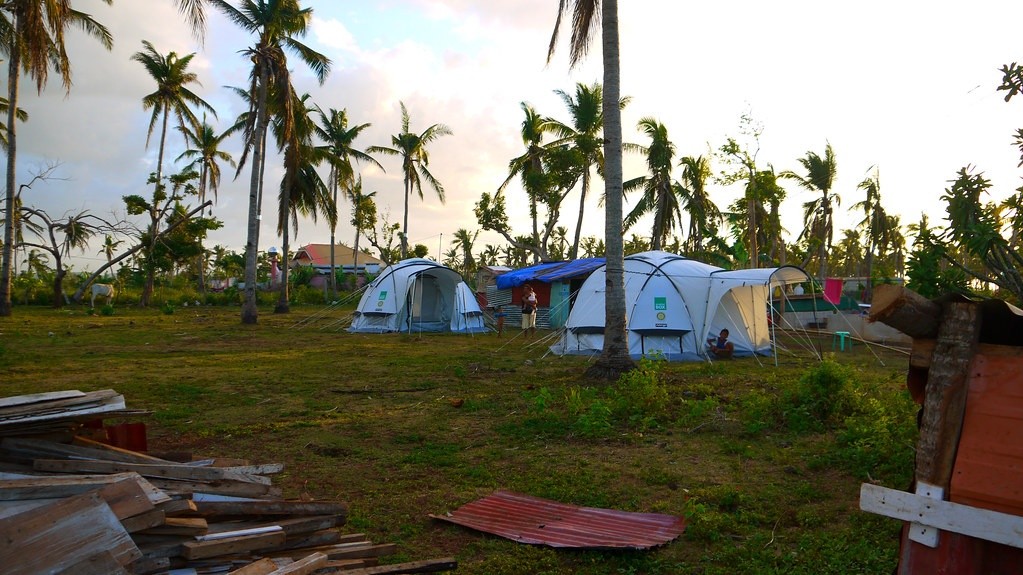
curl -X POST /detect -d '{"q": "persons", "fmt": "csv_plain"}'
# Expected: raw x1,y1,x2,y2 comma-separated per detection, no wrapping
707,329,733,359
495,308,507,338
521,285,538,339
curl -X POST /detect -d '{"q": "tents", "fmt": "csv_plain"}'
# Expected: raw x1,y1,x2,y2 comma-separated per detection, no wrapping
344,258,491,333
548,251,824,365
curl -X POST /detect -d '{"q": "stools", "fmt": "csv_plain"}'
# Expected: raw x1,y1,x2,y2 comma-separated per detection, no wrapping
832,331,852,353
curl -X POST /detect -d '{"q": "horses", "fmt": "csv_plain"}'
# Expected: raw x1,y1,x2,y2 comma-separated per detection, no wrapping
89,283,114,308
